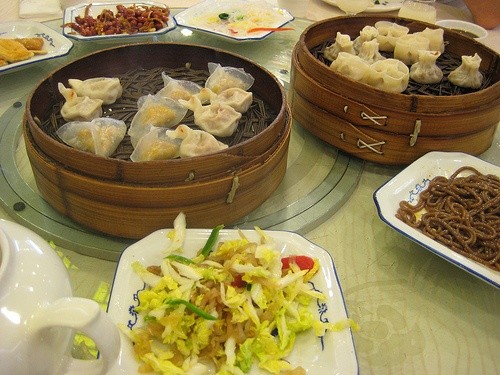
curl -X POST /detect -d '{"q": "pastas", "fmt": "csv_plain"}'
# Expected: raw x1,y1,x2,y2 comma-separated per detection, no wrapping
395,166,500,271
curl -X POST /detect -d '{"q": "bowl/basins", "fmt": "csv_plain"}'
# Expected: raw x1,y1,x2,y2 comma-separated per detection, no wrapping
436,19,488,42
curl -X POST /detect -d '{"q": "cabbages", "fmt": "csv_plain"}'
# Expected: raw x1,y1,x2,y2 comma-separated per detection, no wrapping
118,211,360,375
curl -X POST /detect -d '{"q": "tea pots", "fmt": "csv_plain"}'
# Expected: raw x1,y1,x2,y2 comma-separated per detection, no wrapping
0,219,121,375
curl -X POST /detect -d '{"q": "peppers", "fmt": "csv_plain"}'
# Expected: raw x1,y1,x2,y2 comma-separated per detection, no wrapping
208,9,295,34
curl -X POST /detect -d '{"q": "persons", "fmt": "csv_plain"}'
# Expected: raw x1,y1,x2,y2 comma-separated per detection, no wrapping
434,0,500,30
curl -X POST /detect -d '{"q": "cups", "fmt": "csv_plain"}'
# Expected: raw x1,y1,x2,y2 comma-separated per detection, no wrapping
397,0,438,25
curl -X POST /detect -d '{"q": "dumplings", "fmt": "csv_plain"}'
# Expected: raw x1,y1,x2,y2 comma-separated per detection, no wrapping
57,77,254,158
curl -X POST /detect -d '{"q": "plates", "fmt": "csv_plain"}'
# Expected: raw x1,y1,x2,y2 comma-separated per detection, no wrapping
373,150,500,290
172,0,295,43
321,0,406,12
106,227,359,375
63,2,176,42
0,21,73,76
